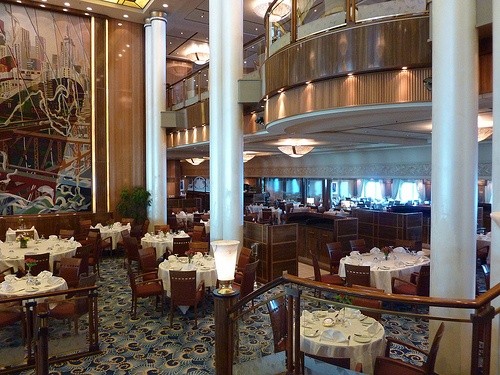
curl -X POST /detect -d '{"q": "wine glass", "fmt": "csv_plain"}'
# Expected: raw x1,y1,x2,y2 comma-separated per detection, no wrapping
204,252,209,259
174,254,178,261
25,270,31,277
327,304,336,312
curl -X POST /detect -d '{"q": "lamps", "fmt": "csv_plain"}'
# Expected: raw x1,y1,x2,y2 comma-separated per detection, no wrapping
211,240,240,292
278,147,315,158
186,158,205,165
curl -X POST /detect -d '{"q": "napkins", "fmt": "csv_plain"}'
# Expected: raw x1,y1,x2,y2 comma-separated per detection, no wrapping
4,274,17,283
322,329,347,342
364,321,379,334
36,271,53,279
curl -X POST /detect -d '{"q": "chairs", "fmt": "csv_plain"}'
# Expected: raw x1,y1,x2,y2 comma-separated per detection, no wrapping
0,204,491,375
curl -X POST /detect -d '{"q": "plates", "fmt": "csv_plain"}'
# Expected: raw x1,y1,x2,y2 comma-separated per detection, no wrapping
342,248,428,270
300,308,380,343
0,276,62,294
163,256,216,270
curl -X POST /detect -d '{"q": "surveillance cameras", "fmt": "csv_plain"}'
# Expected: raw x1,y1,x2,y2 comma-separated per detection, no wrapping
255,117,263,123
424,78,432,84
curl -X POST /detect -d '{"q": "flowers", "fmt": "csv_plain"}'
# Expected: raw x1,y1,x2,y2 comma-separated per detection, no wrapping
16,233,31,241
25,258,38,269
336,294,352,305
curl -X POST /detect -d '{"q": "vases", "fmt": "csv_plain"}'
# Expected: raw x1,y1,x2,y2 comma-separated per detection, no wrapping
20,240,27,247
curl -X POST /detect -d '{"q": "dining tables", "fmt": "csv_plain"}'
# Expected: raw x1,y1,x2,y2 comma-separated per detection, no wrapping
338,250,430,296
92,226,132,251
292,310,386,375
249,205,282,224
0,238,82,277
141,233,190,261
0,276,68,363
159,255,217,301
